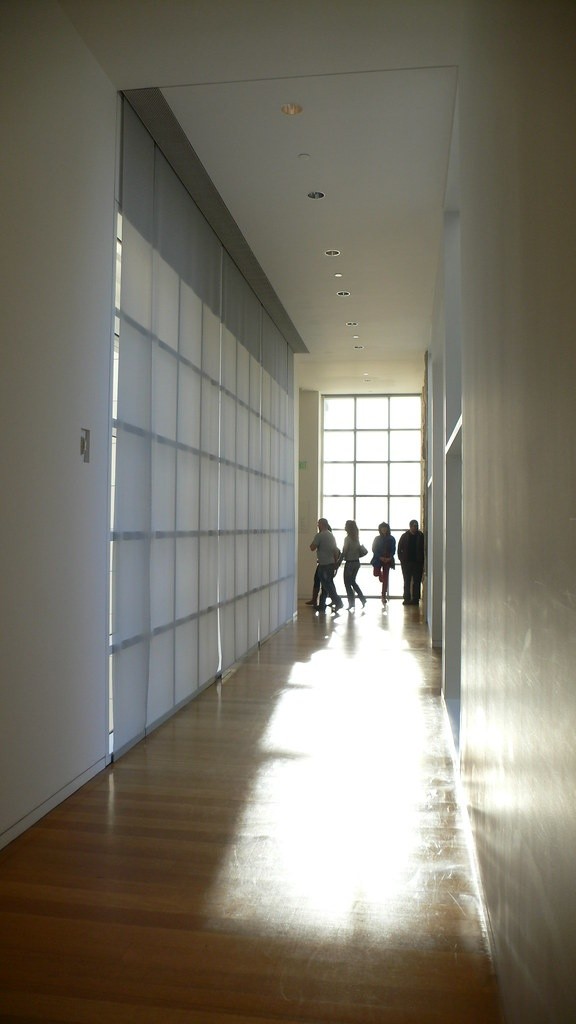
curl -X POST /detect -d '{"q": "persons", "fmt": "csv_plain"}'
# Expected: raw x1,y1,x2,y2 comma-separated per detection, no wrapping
305,518,343,612
398,520,424,604
342,520,368,610
370,522,396,602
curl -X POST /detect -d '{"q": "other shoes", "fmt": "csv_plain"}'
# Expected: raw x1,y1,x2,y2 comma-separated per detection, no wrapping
314,604,326,610
346,599,355,611
409,599,420,605
403,599,410,604
382,596,387,603
361,597,367,609
379,572,383,582
332,601,343,612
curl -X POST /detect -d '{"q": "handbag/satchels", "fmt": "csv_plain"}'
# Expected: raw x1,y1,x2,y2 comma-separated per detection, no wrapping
359,545,368,558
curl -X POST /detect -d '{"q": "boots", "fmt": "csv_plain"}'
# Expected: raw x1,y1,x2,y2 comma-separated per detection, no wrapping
305,587,319,605
